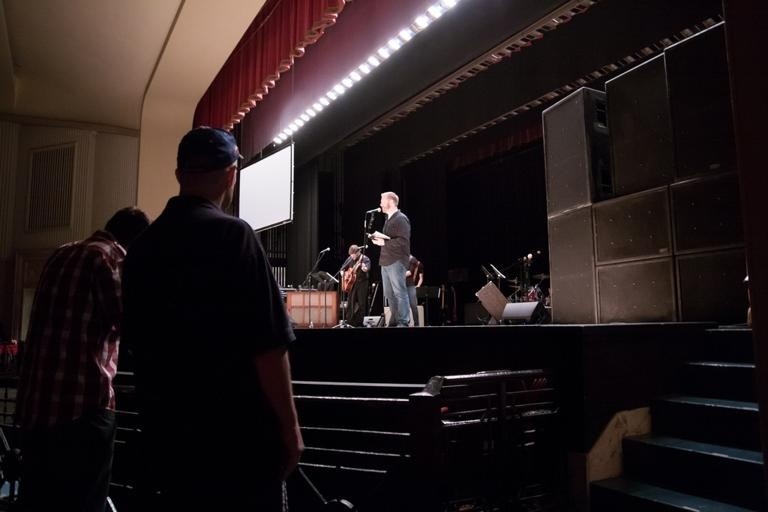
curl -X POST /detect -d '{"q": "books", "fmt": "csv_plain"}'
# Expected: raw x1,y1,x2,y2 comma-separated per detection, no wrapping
366,230,389,245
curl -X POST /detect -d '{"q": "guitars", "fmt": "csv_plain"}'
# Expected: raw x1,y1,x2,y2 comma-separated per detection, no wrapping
341,261,362,293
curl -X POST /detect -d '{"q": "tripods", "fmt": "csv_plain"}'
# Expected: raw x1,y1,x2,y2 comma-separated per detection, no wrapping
330,249,358,329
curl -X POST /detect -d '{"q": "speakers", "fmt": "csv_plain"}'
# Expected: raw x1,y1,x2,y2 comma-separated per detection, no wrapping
501,301,549,325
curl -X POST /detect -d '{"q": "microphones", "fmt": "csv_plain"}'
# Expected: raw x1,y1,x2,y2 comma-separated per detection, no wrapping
355,245,369,251
519,251,541,262
321,246,330,254
326,272,339,283
366,207,383,214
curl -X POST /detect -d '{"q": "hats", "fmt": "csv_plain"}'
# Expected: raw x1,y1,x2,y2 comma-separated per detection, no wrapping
177,125,244,174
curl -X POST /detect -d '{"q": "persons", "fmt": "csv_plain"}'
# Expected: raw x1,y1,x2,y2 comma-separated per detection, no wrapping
339,243,372,328
120,124,307,511
367,190,416,327
416,258,423,327
10,204,153,510
402,249,418,325
